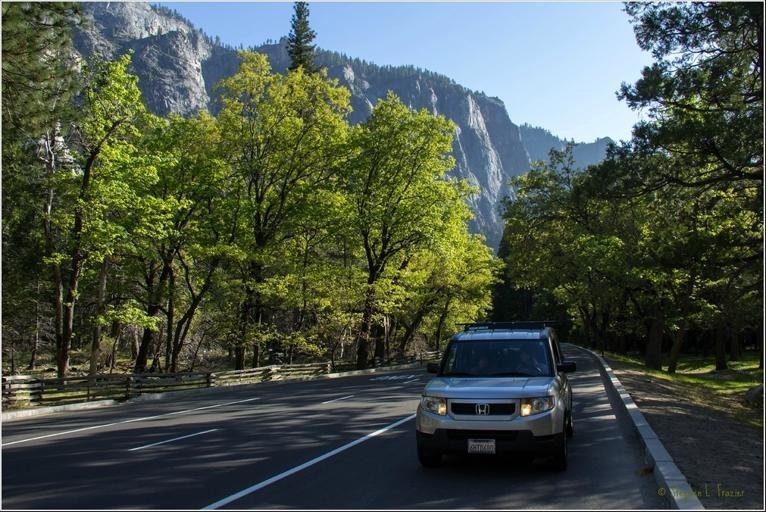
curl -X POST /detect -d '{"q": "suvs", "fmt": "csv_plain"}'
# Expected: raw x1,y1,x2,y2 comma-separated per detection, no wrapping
411,317,577,464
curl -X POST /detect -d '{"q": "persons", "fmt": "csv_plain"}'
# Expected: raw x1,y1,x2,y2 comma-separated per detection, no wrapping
513,350,542,377
471,355,495,373
